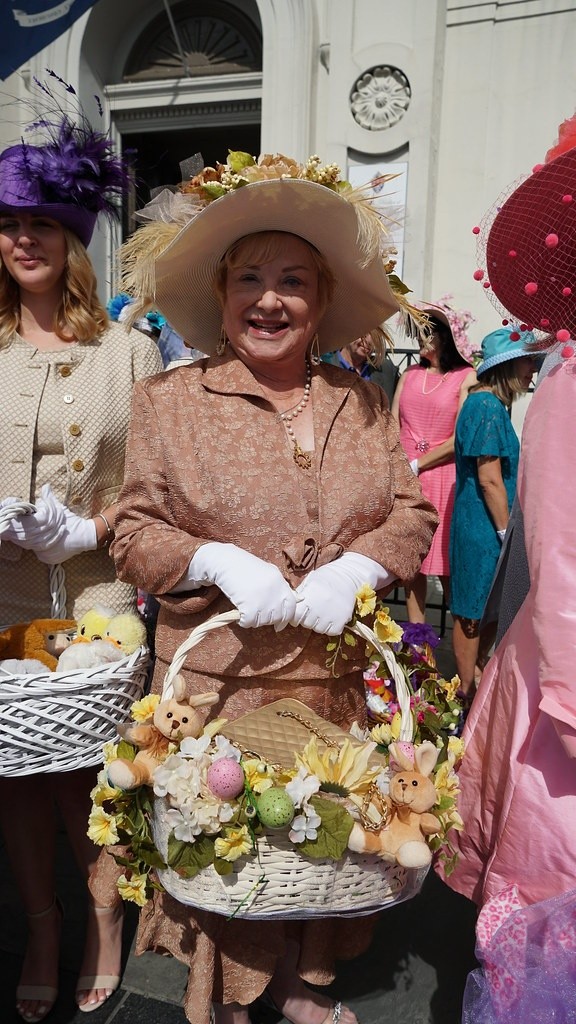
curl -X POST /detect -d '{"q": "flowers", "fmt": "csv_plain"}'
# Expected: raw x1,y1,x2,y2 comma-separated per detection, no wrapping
86,593,468,906
441,297,479,363
186,149,350,194
16,70,133,224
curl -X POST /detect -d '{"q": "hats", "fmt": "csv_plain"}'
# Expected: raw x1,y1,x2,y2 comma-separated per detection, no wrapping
474,116,576,360
0,68,138,249
120,152,435,358
476,324,546,377
408,303,475,368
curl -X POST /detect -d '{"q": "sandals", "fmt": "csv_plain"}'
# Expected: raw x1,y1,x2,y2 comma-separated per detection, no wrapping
13,895,62,1023
73,900,122,1012
269,974,358,1024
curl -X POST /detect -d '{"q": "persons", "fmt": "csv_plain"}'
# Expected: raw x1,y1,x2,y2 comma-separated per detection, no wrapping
0,144,164,1024
109,149,440,1024
331,331,397,404
390,293,480,624
448,326,548,710
437,114,576,1024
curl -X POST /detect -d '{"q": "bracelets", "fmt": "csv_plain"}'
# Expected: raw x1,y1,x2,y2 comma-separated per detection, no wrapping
93,513,111,547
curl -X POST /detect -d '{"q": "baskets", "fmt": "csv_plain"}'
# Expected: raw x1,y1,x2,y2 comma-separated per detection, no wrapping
150,605,435,919
0,496,151,778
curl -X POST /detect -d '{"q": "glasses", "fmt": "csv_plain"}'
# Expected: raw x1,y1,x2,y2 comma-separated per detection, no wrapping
423,324,442,338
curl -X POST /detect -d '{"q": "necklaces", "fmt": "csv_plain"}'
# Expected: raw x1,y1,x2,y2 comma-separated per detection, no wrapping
279,360,311,469
423,366,449,394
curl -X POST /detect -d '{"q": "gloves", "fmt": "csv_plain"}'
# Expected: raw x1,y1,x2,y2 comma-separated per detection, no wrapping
37,500,97,565
292,550,394,638
0,494,67,552
171,542,296,633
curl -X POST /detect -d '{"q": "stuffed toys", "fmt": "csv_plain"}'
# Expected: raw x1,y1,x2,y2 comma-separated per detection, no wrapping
0,605,146,677
109,675,219,791
347,740,441,869
